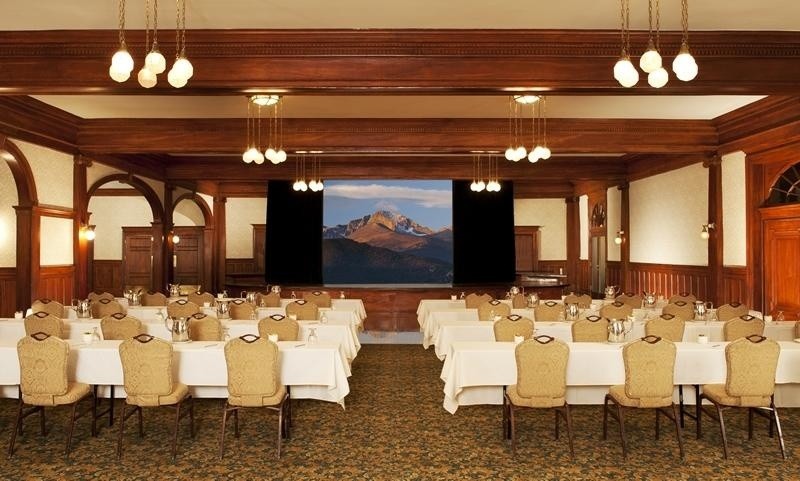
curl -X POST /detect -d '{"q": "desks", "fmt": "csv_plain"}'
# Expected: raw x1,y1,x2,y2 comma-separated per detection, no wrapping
441,340,800,414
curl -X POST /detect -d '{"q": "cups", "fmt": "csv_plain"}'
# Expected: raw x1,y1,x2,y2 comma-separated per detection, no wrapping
764,313,774,324
13,310,24,323
451,293,457,301
289,312,298,321
488,309,502,323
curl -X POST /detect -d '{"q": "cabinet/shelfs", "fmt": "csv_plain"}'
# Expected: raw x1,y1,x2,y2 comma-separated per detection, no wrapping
120,225,207,294
513,223,542,274
755,200,800,324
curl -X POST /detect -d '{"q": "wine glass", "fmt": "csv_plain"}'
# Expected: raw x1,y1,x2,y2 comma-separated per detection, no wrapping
459,291,466,300
307,326,318,346
777,310,787,325
340,289,346,300
320,309,329,324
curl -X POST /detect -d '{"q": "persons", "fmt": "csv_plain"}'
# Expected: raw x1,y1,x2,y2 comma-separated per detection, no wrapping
592,203,605,227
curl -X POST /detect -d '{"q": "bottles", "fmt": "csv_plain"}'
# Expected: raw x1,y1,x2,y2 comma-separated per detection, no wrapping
291,290,297,300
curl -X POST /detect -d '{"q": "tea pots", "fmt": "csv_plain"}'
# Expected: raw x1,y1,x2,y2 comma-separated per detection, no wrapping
71,282,281,344
507,284,714,344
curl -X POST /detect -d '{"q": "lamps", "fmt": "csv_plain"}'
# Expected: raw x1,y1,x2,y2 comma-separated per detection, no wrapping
108,0,198,89
615,229,626,245
242,92,288,167
506,95,552,162
613,0,699,88
293,149,324,192
701,220,716,239
468,152,503,193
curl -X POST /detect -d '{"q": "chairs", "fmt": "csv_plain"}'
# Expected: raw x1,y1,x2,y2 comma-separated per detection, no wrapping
8,285,332,461
466,288,766,345
696,335,788,462
501,334,576,460
601,334,685,457
